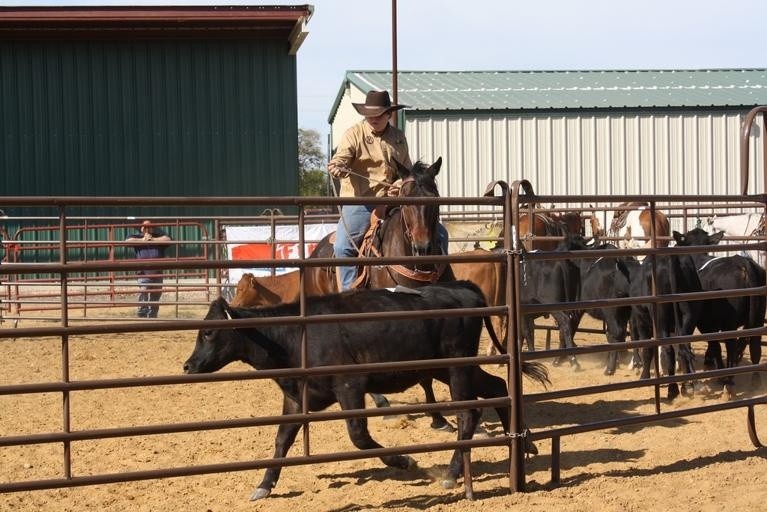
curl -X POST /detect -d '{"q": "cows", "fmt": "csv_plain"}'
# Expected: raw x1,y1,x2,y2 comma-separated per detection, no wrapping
231,269,301,309
450,197,767,405
181,277,551,504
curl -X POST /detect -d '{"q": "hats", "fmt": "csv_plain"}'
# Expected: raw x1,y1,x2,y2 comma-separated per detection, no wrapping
352,90,412,117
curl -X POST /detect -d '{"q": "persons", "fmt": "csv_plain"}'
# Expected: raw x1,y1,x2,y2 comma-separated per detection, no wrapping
124,218,172,319
326,91,450,297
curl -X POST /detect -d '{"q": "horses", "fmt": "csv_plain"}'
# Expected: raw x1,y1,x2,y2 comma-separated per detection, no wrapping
302,158,485,434
